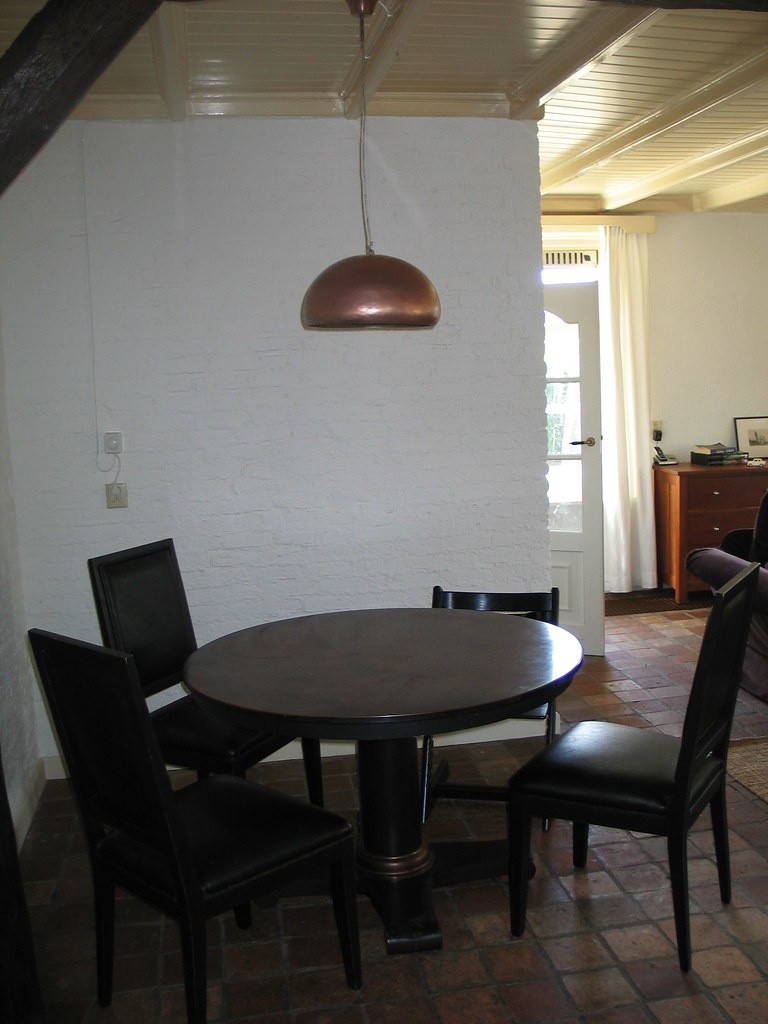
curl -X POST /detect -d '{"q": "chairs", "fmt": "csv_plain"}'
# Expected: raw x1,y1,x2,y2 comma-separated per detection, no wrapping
509,560,763,974
86,539,326,932
28,628,361,1024
419,586,560,835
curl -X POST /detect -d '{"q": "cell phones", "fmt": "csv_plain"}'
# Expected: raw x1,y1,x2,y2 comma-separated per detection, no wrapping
654,446,667,460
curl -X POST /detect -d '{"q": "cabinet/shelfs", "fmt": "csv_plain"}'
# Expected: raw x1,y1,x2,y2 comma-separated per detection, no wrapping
653,459,768,604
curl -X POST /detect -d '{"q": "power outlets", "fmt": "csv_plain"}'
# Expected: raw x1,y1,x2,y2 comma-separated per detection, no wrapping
651,420,662,442
104,483,128,509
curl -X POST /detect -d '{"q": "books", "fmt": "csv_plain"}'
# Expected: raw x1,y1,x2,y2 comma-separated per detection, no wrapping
691,443,749,466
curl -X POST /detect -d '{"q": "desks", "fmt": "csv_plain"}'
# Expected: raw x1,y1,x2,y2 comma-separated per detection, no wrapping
179,607,584,957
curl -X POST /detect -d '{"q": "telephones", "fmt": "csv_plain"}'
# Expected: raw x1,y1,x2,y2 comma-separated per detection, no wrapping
653,446,679,465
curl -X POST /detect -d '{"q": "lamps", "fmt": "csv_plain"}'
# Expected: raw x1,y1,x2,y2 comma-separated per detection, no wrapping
299,0,442,332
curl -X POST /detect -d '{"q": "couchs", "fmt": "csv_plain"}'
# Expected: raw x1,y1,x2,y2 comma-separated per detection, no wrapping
685,490,768,708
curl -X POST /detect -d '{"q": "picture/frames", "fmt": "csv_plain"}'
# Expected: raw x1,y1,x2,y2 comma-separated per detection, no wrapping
733,416,768,460
